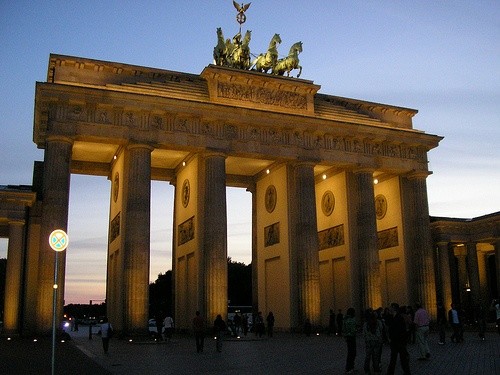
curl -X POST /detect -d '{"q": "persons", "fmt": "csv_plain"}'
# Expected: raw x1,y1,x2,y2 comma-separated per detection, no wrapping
74,316,79,332
304,319,312,336
435,303,447,345
266,312,274,337
155,309,174,344
213,315,226,353
489,300,500,334
448,304,465,343
100,317,113,354
193,311,204,353
475,303,486,341
327,298,431,375
226,312,264,339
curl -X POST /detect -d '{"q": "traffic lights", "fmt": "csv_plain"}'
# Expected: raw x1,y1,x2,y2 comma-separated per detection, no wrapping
64,315,67,318
68,317,72,320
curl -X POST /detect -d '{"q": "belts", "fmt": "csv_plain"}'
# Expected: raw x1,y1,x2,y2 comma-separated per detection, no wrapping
418,325,429,327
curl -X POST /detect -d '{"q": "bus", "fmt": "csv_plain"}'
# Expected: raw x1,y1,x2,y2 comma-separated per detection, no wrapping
227,306,258,331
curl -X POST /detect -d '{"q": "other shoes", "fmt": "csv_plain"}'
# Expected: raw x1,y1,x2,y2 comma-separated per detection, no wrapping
417,358,426,361
426,353,430,359
437,342,444,345
346,368,358,372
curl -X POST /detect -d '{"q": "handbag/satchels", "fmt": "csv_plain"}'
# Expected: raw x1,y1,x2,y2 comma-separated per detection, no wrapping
107,323,113,338
380,319,389,344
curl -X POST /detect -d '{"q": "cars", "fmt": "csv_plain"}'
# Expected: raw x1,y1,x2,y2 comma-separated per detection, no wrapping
148,319,167,334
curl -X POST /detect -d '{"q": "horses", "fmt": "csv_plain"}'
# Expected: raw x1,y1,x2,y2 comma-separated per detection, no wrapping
214,27,252,70
256,33,282,75
273,41,303,78
